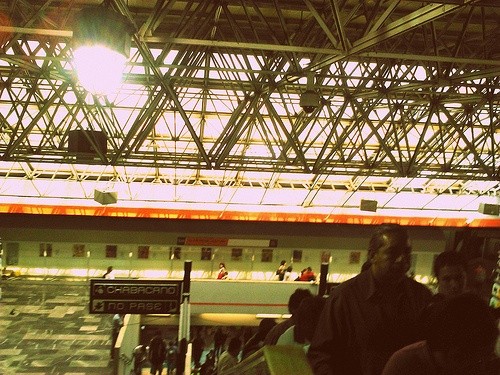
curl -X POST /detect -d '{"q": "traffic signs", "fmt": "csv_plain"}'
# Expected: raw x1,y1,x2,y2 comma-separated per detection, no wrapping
90,279,181,315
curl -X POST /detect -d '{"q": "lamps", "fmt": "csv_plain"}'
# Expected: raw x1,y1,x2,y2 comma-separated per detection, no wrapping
72,8,134,92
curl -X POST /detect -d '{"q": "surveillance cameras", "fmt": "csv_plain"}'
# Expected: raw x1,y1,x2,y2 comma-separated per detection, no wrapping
299,91,319,113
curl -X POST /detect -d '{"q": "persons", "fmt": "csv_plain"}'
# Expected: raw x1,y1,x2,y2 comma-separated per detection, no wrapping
103,261,327,375
307,223,500,375
